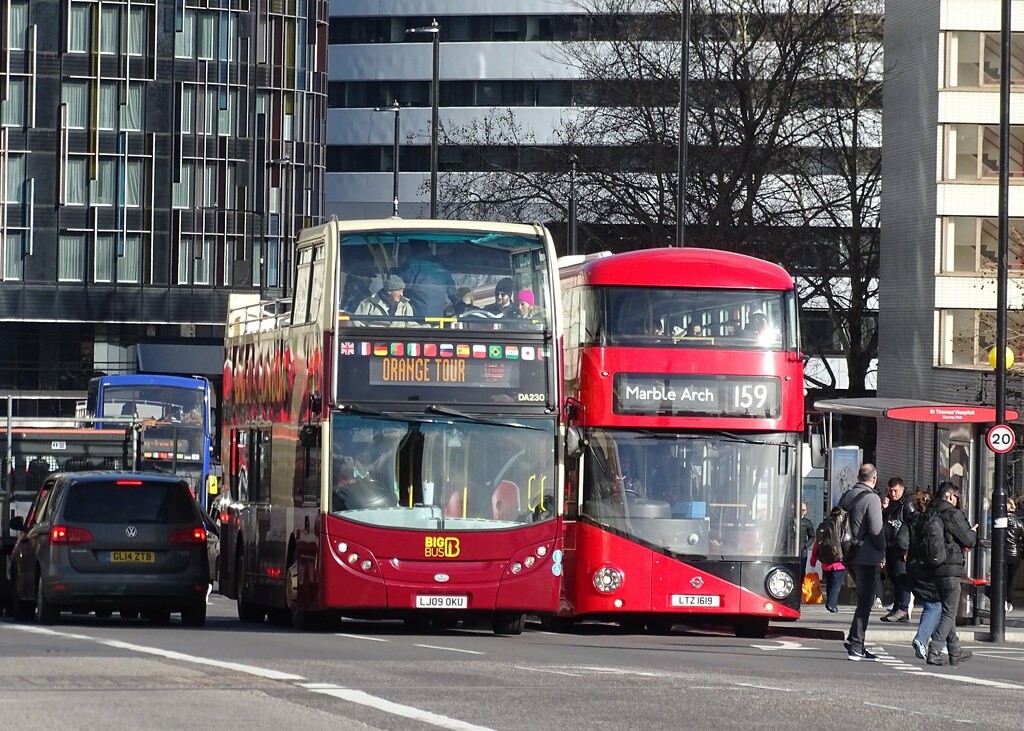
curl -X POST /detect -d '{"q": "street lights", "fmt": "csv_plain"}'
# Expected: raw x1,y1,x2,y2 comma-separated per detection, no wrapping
405,18,440,218
374,99,400,216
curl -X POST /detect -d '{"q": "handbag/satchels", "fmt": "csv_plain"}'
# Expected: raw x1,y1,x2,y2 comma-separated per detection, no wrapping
801,569,824,604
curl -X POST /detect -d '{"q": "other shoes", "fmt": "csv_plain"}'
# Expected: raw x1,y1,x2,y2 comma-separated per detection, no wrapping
1005,602,1013,615
826,605,837,612
871,602,884,611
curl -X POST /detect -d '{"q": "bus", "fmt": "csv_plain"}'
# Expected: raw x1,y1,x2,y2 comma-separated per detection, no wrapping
85,375,218,511
0,425,142,550
220,213,583,635
470,247,833,637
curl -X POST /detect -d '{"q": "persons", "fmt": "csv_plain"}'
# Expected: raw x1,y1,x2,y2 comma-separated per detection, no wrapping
27,456,50,473
688,323,702,337
400,239,456,325
809,507,846,613
839,463,886,661
482,277,513,316
906,481,975,664
592,453,647,501
651,443,684,503
121,402,137,415
879,478,909,623
454,287,482,314
744,310,768,331
652,319,662,335
801,503,815,585
1005,498,1024,612
341,448,380,486
502,290,542,330
341,265,377,313
353,274,419,328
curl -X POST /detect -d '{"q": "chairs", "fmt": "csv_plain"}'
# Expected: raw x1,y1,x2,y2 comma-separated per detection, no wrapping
485,480,521,521
440,478,478,515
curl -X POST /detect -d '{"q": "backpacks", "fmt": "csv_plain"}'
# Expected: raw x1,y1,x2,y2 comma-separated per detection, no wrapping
916,506,953,569
818,488,874,564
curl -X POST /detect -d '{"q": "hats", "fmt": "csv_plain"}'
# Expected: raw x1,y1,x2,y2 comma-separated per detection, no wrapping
516,291,534,306
352,266,378,278
495,277,514,298
383,275,405,292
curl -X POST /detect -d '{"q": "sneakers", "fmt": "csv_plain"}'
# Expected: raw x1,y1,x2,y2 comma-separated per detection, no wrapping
844,638,879,662
912,638,928,659
950,650,972,666
880,608,909,623
927,652,947,666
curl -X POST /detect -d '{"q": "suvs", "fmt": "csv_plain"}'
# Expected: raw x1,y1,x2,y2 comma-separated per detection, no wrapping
8,469,210,626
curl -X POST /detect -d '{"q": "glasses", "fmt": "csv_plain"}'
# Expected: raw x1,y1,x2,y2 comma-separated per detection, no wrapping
496,291,507,297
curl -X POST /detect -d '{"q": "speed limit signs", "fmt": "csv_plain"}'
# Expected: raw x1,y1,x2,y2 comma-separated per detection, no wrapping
987,425,1015,454
